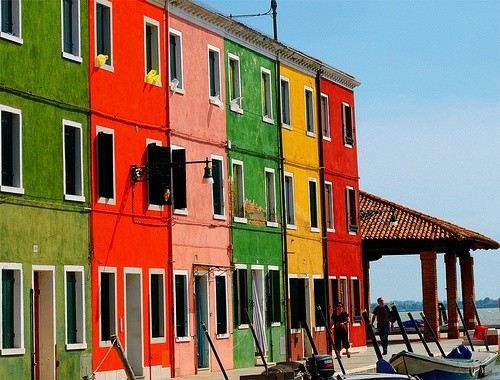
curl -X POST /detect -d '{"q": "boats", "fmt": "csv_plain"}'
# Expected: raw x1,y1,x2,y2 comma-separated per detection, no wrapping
306,343,499,380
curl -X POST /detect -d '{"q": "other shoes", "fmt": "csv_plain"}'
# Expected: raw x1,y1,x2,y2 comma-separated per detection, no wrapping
335,356,341,359
382,349,387,355
347,354,350,358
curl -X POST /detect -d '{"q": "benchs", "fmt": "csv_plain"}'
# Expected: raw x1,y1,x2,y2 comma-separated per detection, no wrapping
375,325,466,336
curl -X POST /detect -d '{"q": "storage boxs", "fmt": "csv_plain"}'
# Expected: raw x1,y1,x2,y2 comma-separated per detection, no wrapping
485,329,500,345
307,354,335,377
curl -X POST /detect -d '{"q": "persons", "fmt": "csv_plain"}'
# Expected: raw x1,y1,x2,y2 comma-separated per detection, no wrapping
371,297,393,355
329,303,350,359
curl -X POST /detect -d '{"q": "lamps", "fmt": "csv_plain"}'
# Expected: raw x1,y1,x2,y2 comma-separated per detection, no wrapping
131,158,214,188
361,208,398,226
